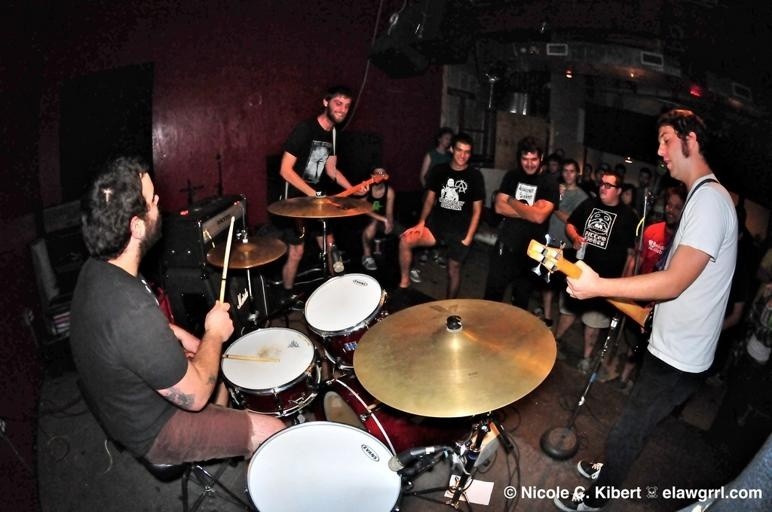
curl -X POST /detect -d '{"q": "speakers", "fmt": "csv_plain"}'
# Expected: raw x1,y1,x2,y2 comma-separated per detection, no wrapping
159,268,266,353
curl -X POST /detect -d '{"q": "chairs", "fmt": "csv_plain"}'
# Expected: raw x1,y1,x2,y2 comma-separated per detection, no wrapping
75,379,256,510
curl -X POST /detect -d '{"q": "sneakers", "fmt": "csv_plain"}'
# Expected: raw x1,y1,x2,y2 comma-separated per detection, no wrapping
408,253,448,283
279,293,304,310
362,256,377,270
579,359,632,388
578,460,603,479
554,493,600,512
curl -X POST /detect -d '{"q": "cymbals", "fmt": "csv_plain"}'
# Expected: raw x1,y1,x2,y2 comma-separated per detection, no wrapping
353,299,557,418
207,236,287,269
268,195,372,218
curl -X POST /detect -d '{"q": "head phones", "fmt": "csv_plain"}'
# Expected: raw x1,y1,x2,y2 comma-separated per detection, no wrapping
656,117,696,135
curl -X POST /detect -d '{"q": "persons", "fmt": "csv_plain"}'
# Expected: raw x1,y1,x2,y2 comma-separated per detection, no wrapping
357,108,772,511
272,86,372,313
62,154,297,476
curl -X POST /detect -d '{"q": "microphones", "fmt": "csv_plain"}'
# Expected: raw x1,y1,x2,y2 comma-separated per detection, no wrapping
388,445,447,472
646,163,667,206
331,248,344,273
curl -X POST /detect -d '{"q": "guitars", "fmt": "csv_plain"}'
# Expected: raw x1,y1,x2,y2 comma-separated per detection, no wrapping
527,234,654,358
296,168,389,233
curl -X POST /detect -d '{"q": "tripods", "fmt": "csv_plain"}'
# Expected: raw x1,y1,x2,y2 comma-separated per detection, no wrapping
399,420,492,512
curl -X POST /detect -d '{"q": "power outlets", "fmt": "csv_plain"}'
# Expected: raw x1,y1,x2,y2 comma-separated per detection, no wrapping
18,307,35,329
0,418,8,440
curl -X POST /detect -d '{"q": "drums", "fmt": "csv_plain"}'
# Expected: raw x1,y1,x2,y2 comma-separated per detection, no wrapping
244,420,404,512
322,375,472,455
302,271,391,375
221,326,323,418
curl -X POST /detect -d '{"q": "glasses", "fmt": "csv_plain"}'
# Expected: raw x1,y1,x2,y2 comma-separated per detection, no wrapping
599,180,616,189
